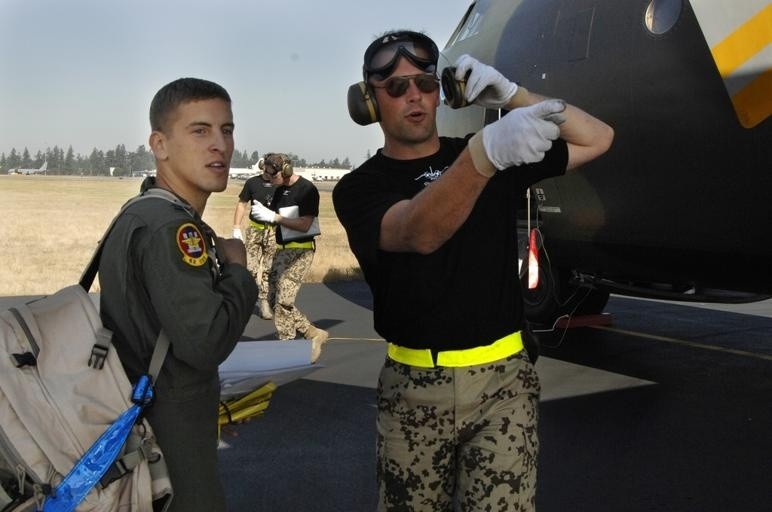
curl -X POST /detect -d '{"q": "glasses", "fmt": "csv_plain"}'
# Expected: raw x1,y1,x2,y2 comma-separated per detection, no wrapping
362,31,441,82
372,73,440,99
264,160,283,176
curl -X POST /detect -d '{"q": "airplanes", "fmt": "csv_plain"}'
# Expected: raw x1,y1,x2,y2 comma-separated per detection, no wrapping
436,1,772,327
8,162,48,175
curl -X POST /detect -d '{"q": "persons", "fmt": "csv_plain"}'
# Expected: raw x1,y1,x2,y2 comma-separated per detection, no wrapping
332,26,618,512
250,152,330,365
231,151,280,320
96,75,261,512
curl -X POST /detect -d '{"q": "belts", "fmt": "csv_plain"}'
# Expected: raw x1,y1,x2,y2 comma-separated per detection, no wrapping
249,220,273,231
386,326,525,370
276,240,314,250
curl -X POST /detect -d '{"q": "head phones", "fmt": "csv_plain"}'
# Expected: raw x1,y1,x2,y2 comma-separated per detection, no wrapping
256,158,265,171
346,31,472,126
278,152,294,179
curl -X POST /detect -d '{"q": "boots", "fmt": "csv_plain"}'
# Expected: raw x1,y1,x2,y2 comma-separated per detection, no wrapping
258,299,274,321
302,323,330,365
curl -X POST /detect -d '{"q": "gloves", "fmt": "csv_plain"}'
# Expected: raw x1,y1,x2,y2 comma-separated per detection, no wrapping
452,53,529,112
232,228,244,243
250,199,276,225
468,98,567,178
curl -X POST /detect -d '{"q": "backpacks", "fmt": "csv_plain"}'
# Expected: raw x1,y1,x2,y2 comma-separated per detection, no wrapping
0,187,204,512
